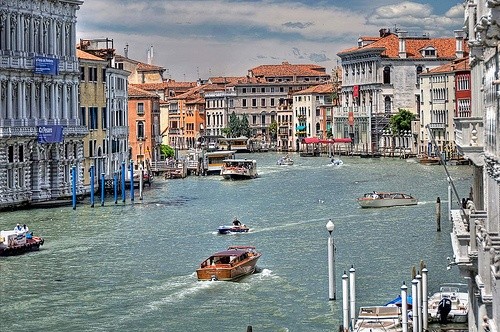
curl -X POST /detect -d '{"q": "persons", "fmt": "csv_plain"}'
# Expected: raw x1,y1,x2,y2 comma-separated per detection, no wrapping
13,223,21,231
20,224,28,233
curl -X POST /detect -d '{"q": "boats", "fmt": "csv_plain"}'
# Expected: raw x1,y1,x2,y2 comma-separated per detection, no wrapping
220,159,258,180
424,282,470,323
197,246,262,284
344,293,424,332
165,133,470,179
0,231,44,256
129,174,153,182
217,225,249,234
357,191,417,208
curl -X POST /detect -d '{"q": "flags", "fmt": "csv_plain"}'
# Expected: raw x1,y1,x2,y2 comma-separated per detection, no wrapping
36,125,63,145
34,56,60,76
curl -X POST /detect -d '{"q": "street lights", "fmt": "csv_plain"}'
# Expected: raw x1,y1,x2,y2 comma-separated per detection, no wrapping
326,219,336,301
448,176,454,221
